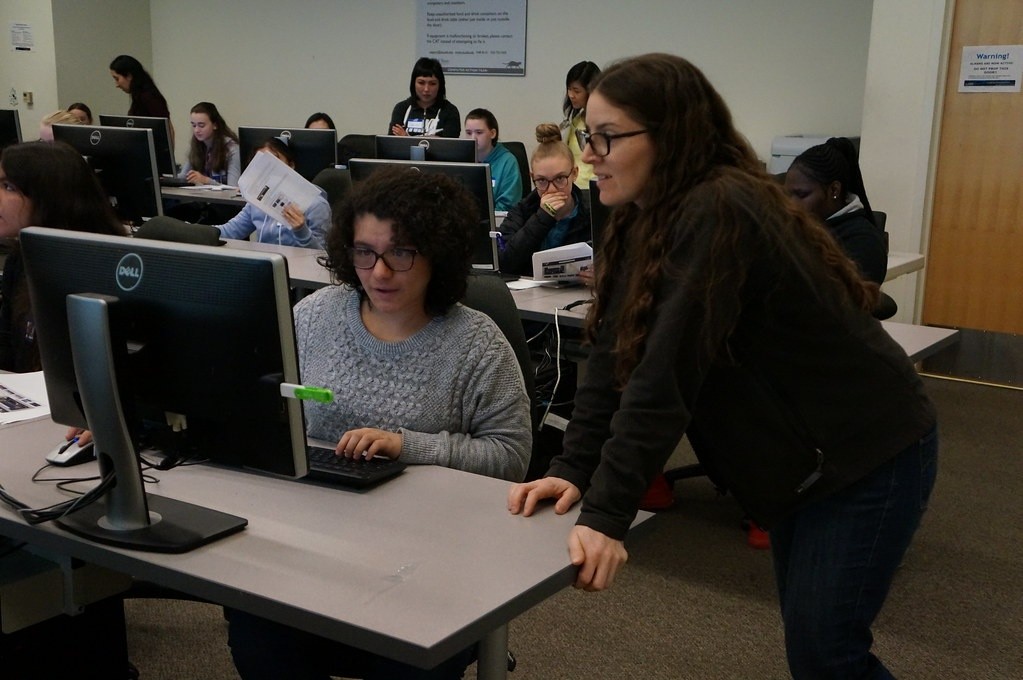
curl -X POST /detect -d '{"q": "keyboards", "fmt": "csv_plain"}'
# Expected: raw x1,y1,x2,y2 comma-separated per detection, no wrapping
159,177,196,186
307,445,406,489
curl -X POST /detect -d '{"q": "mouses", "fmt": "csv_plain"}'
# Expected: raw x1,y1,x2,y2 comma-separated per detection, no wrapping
45,435,97,467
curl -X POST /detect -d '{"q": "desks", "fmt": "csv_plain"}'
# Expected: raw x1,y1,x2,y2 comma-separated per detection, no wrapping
1,182,962,680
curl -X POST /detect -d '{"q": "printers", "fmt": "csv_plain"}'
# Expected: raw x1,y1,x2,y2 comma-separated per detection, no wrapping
768,134,831,176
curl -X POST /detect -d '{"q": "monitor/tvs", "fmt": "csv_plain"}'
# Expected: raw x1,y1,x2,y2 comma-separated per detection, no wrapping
0,110,501,554
587,179,613,261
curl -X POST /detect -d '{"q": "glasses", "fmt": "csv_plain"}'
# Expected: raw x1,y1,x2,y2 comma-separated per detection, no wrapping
532,167,574,191
351,247,419,272
575,127,649,157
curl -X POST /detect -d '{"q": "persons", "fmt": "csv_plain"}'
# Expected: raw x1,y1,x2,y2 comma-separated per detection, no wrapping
210,137,332,249
109,55,175,152
388,57,461,138
290,164,532,483
498,123,590,275
0,138,132,447
557,60,601,205
508,52,938,680
177,102,240,186
304,111,336,130
463,108,522,227
68,103,93,124
785,136,889,290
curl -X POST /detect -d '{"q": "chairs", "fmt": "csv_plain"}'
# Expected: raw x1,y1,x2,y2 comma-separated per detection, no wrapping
499,143,532,198
311,168,354,227
459,270,550,671
130,215,222,245
336,132,376,163
